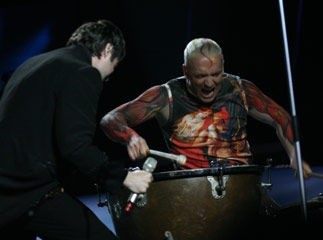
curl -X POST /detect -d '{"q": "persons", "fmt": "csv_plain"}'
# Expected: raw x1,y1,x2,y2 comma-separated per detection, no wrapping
0,16,155,239
90,37,315,197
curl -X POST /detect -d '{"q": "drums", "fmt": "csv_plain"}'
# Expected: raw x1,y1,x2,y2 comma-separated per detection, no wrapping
98,164,276,240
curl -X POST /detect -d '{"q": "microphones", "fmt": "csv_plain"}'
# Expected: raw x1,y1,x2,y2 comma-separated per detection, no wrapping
125,157,157,214
